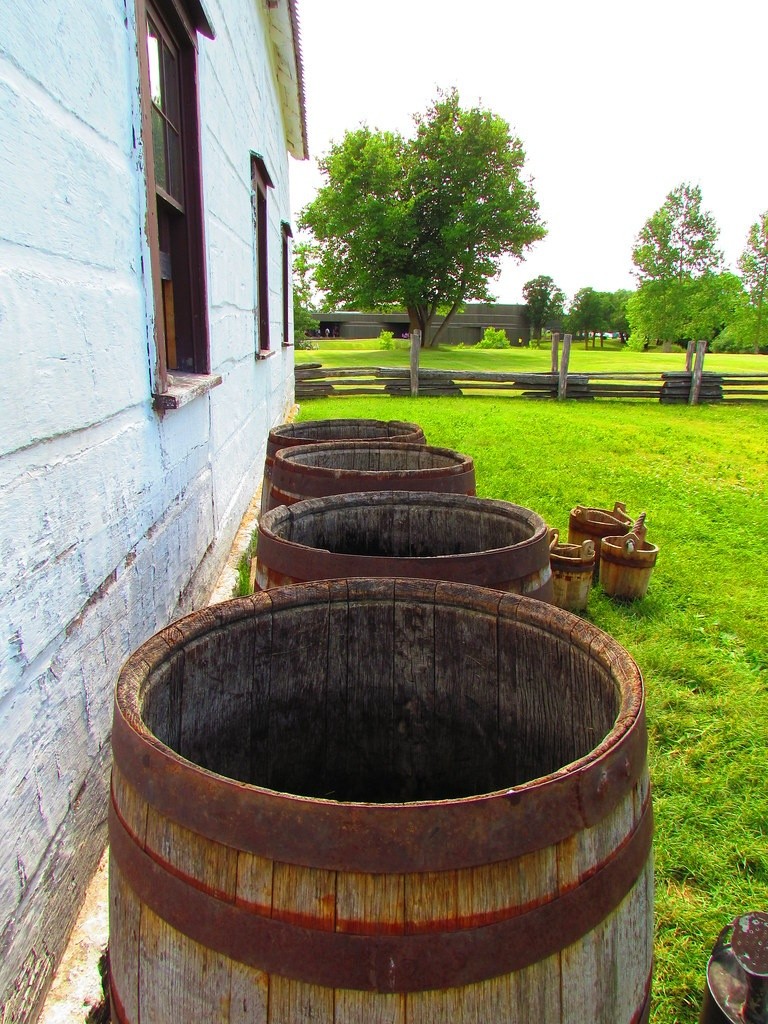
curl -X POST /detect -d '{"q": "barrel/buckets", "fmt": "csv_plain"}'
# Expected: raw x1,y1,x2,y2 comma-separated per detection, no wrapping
548,529,596,612
101,576,654,1024
600,533,660,603
255,490,554,608
262,418,427,516
568,502,636,562
268,442,478,514
